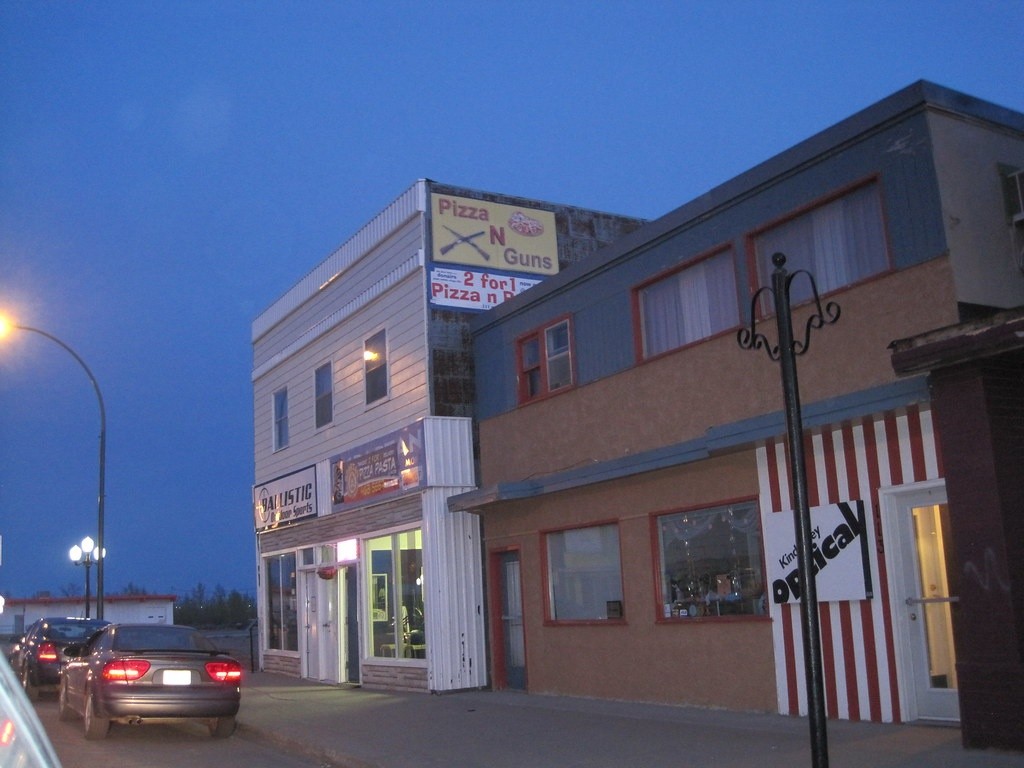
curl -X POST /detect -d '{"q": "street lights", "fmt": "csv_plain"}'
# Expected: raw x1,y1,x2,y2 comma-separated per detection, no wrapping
69,536,107,618
0,320,107,619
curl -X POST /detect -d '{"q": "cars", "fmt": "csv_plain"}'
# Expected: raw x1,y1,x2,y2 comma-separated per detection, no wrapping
7,617,113,698
374,605,426,658
60,623,242,740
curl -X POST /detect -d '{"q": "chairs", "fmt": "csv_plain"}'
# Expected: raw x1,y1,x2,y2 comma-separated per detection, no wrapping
380,646,393,657
404,646,414,658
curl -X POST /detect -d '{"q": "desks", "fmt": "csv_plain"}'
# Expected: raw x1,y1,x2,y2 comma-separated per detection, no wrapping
382,644,426,658
